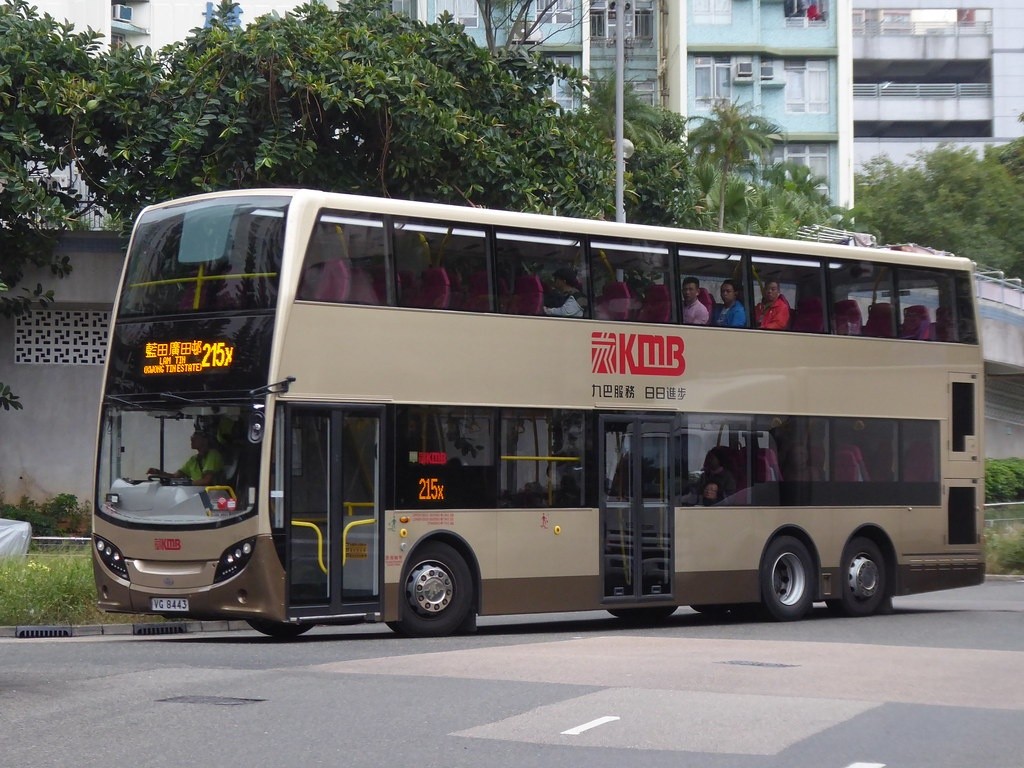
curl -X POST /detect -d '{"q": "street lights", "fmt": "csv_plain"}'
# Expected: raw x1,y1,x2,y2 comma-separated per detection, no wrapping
613,138,634,223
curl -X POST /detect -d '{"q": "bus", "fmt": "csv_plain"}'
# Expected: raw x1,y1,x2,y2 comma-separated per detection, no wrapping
87,185,986,636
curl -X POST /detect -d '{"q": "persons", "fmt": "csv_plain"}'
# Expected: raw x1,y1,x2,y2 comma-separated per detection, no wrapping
693,482,723,507
682,277,709,325
755,278,789,330
712,279,747,327
546,453,580,507
146,429,226,486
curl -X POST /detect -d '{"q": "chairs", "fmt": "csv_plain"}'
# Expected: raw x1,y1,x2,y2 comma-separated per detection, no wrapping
181,255,952,343
219,445,243,482
612,440,935,495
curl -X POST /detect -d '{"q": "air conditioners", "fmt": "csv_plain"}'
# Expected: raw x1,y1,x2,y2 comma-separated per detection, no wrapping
511,22,540,41
760,67,774,78
737,62,753,75
111,4,134,22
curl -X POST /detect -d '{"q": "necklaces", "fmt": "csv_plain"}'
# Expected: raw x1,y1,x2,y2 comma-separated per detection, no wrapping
679,446,738,505
543,268,589,317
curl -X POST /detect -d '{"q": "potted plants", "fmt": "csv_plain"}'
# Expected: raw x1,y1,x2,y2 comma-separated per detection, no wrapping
982,457,1024,576
